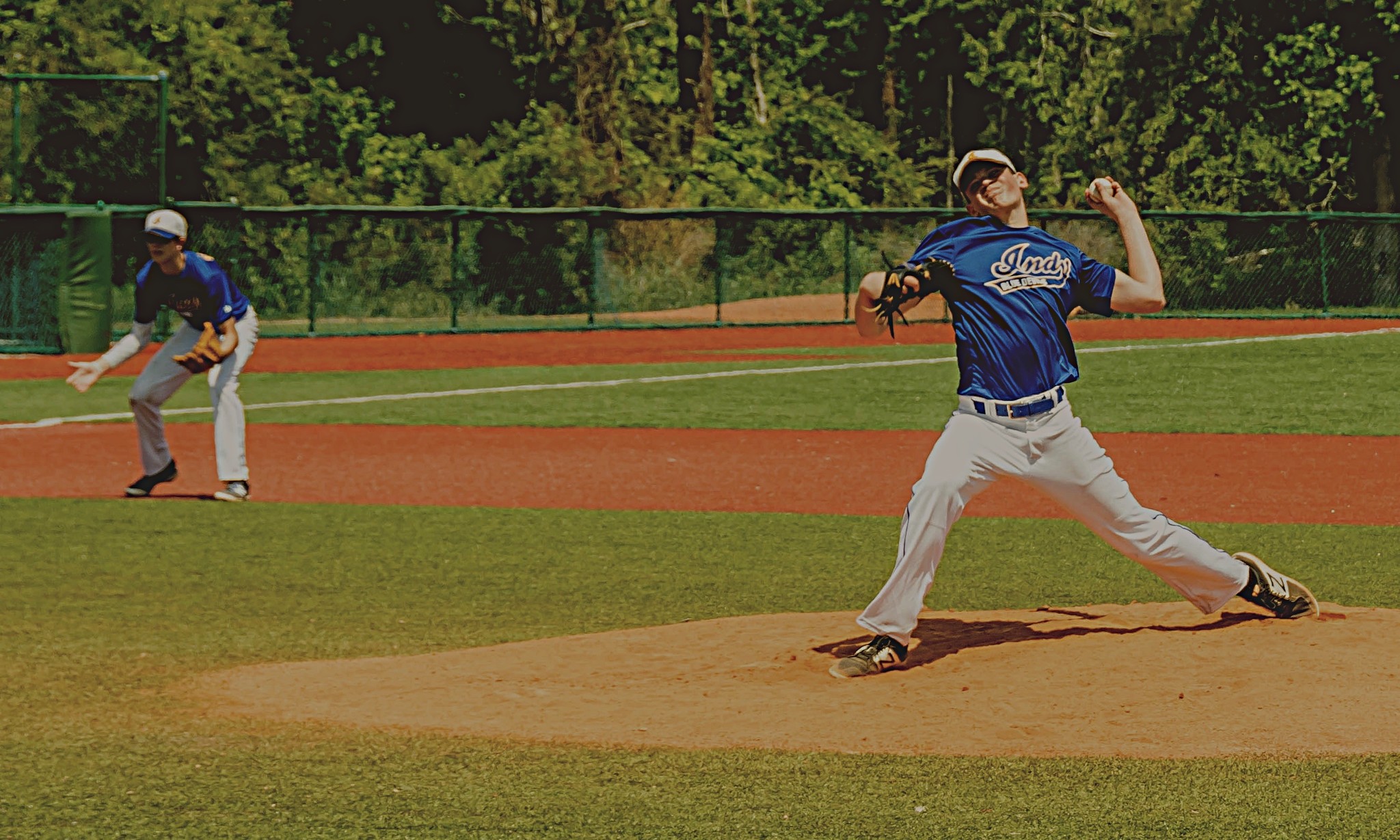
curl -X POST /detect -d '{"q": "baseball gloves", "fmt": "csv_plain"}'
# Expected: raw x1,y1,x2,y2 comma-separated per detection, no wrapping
172,321,226,374
860,248,955,341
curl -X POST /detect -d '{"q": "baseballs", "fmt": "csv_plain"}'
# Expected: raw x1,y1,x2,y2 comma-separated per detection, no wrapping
1088,178,1114,203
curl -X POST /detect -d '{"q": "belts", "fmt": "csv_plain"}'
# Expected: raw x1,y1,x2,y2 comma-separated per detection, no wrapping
970,387,1065,418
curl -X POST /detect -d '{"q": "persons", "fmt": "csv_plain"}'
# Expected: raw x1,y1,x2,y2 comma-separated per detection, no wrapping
61,209,263,498
819,149,1326,676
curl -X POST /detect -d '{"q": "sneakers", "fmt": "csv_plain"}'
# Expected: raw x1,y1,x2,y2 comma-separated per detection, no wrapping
125,459,177,496
214,480,250,503
828,632,908,680
1231,549,1319,620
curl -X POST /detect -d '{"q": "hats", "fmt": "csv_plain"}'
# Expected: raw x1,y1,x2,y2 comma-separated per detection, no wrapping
954,147,1018,192
134,209,187,243
